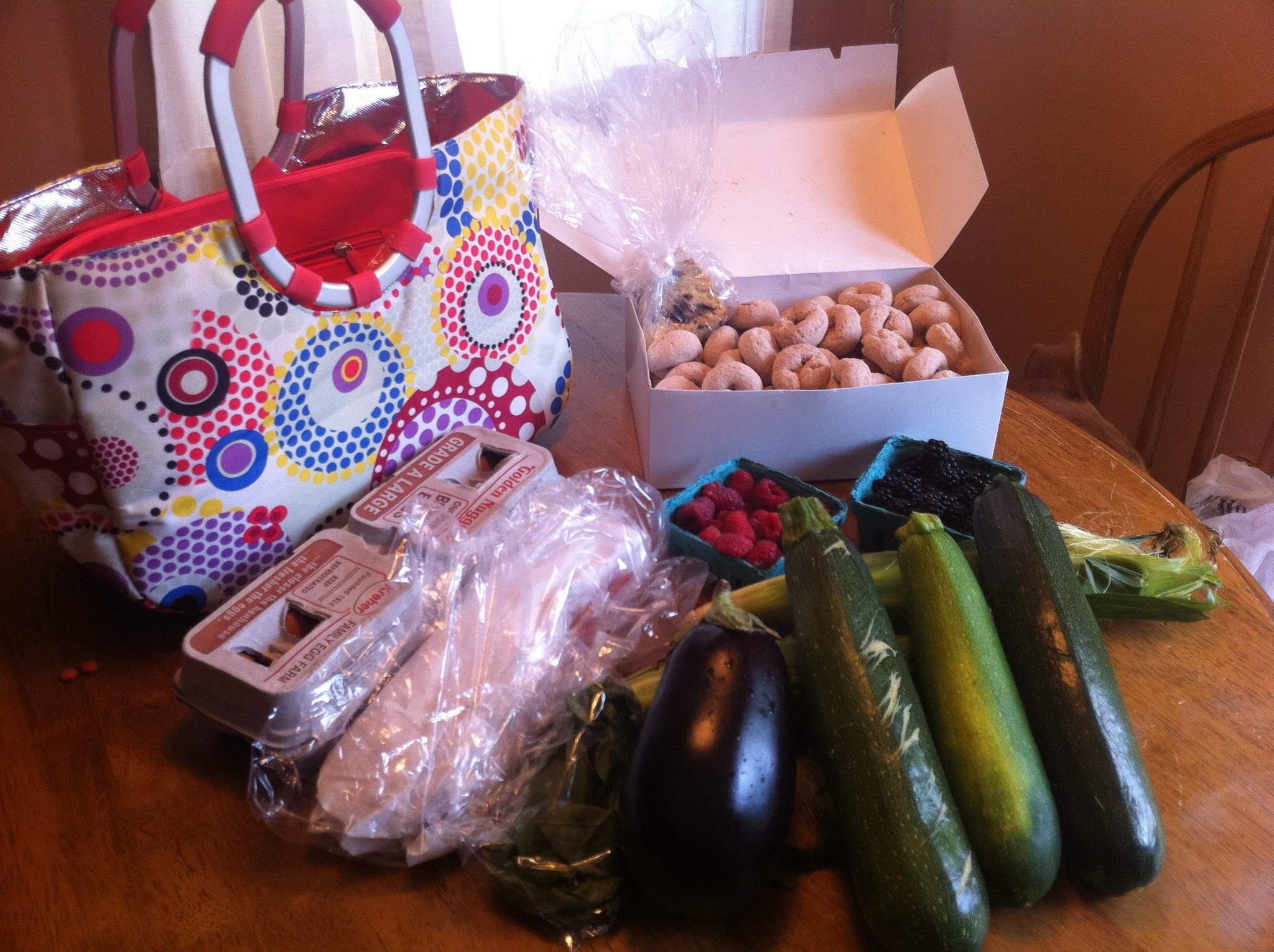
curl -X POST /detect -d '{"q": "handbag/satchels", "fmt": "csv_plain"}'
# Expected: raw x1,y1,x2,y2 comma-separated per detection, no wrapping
1185,454,1274,602
0,0,575,615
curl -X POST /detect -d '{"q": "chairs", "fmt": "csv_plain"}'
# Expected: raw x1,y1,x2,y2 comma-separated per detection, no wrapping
1077,113,1274,502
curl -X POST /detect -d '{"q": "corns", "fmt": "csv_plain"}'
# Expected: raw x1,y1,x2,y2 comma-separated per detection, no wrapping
620,538,1208,714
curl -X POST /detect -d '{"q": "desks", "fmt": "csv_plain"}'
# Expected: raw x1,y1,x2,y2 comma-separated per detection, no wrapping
0,292,1274,952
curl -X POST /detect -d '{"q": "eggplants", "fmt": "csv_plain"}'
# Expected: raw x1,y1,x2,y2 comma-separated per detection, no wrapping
619,573,797,947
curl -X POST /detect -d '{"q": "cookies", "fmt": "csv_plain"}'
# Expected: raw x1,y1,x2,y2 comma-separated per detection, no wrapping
645,278,975,392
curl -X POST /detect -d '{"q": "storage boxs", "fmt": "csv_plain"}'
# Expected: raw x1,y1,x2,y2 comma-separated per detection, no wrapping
536,42,1011,489
851,435,1027,555
658,457,847,597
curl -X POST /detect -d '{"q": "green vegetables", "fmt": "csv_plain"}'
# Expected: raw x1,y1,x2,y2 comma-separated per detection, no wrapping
474,672,648,940
